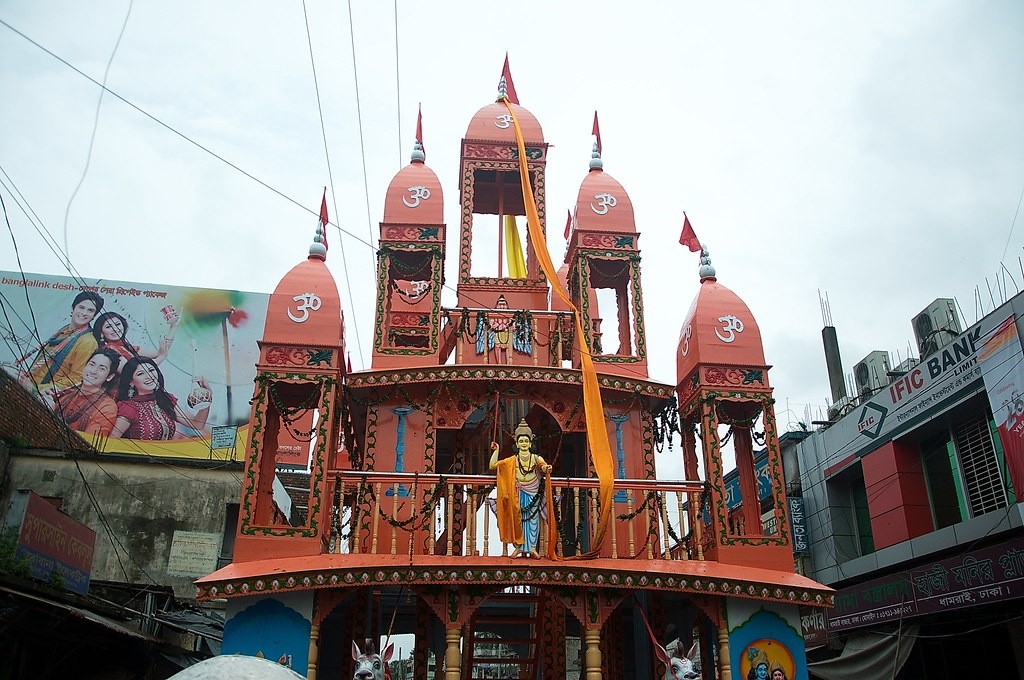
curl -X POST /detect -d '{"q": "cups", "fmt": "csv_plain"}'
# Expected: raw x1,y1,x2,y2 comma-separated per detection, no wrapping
159,305,180,324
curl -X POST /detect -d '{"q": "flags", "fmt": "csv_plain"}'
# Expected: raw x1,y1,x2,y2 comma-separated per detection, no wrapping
592,114,602,154
416,112,425,155
679,217,702,252
318,197,328,248
497,57,519,104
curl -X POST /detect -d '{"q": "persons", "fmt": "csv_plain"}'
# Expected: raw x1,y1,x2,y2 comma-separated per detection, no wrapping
18,291,104,412
489,434,552,558
93,312,135,403
53,347,117,438
491,294,514,364
109,356,214,440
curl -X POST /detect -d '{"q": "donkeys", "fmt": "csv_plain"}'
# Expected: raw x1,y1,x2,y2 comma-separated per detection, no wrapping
350,640,394,680
654,642,702,680
